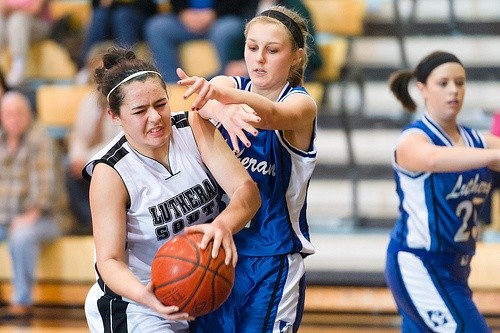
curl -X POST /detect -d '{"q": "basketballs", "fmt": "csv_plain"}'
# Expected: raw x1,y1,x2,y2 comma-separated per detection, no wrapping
151,233,235,317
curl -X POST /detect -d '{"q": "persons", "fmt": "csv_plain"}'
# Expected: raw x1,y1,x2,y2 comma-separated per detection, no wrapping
175,5,320,333
384,52,500,333
0,0,316,323
80,45,263,333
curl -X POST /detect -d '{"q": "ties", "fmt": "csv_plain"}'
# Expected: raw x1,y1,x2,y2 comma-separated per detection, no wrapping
90,108,105,146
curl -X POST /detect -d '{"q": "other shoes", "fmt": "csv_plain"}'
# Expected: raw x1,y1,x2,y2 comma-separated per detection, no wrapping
0,303,32,325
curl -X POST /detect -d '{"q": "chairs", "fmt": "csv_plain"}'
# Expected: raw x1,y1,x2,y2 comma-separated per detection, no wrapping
0,0,456,285
